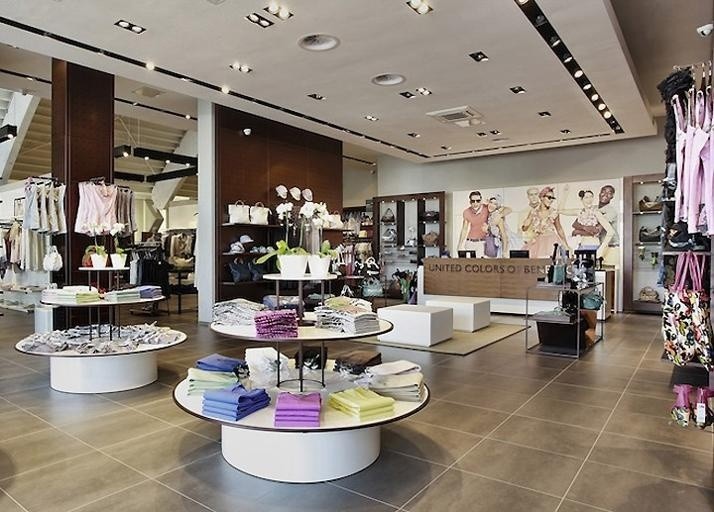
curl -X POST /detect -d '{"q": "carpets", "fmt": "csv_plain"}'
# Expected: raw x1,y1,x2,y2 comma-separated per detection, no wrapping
350,323,531,356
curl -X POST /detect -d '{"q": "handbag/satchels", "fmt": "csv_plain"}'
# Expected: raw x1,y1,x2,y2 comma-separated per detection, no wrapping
381,208,395,222
662,253,713,372
383,229,396,243
229,257,268,283
422,231,438,246
423,211,439,220
387,273,417,303
639,287,659,303
547,246,564,284
43,247,63,272
582,293,603,309
229,200,250,225
363,279,384,297
251,202,271,225
640,227,660,240
639,196,661,211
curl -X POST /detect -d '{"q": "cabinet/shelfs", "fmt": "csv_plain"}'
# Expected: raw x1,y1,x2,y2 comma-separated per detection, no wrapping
622,173,668,312
218,221,290,296
521,277,606,360
369,190,446,285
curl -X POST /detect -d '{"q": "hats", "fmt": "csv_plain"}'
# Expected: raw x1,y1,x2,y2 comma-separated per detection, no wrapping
290,187,301,201
302,188,313,202
276,185,287,199
538,187,554,197
240,234,253,243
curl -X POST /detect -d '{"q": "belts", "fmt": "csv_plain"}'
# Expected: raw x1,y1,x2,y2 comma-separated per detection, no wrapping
467,238,484,242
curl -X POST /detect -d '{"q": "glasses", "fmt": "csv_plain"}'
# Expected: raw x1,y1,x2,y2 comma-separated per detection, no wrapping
471,200,480,203
545,195,555,199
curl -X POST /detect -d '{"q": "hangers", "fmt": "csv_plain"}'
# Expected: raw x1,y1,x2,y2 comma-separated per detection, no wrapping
669,59,714,107
24,176,65,198
130,243,165,261
78,177,134,200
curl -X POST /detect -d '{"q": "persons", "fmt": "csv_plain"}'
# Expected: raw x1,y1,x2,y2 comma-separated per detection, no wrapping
570,184,621,269
515,185,540,245
555,183,614,259
520,185,571,258
480,193,508,258
455,191,512,258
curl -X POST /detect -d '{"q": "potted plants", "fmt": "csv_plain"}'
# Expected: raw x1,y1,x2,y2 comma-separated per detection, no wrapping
84,222,129,268
256,230,339,278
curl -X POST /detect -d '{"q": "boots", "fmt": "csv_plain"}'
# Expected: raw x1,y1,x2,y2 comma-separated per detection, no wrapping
693,386,713,429
670,384,692,426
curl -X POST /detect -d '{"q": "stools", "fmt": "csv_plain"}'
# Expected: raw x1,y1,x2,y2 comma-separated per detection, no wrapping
370,297,491,347
